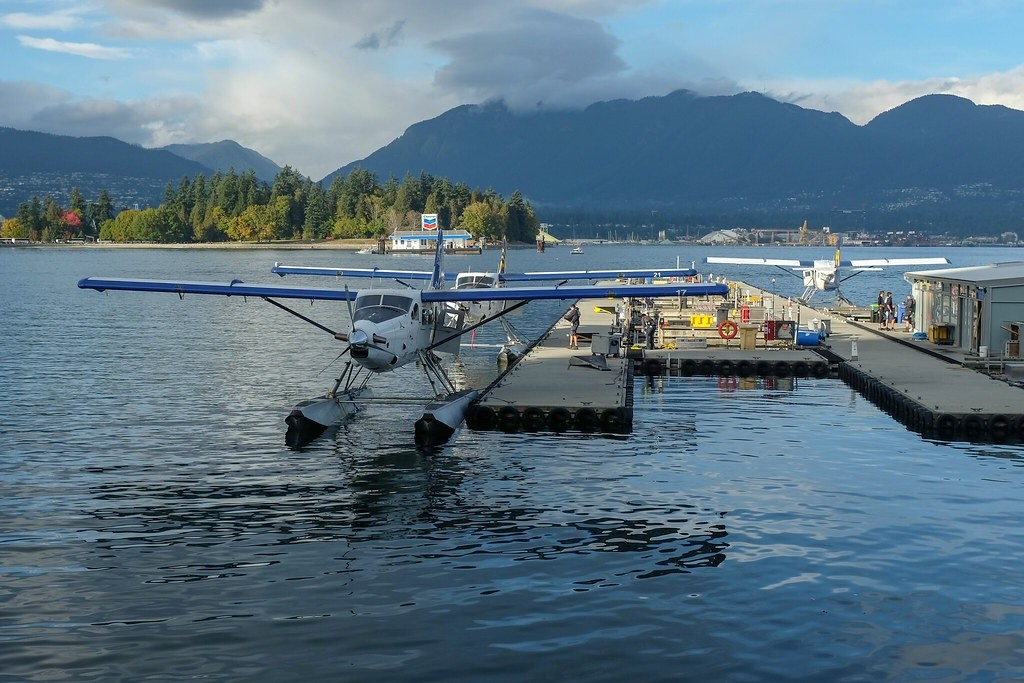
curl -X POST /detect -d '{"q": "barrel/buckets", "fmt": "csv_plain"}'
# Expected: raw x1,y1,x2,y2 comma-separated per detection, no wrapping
980,346,987,357
797,333,819,345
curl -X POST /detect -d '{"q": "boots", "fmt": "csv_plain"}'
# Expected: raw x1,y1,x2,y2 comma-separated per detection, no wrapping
902,327,909,332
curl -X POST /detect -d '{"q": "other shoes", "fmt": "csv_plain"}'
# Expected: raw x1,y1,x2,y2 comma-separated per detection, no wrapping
573,346,578,350
879,328,885,330
567,345,572,349
885,327,890,331
892,327,894,330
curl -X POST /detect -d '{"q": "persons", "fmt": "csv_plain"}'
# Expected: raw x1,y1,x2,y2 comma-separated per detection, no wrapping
641,314,656,350
901,294,916,333
883,292,896,331
564,304,581,350
878,291,886,331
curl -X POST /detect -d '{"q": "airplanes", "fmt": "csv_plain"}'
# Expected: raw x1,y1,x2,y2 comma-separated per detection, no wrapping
701,249,952,308
77,230,730,455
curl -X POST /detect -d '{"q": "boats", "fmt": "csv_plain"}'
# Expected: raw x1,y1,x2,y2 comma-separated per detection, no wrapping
570,248,583,254
356,248,373,254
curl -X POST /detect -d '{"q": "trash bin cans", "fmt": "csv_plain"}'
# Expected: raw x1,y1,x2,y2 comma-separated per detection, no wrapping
681,297,688,310
716,308,729,327
870,304,898,323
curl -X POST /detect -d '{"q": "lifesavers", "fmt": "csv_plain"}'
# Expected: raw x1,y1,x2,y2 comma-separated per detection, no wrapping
718,320,738,339
